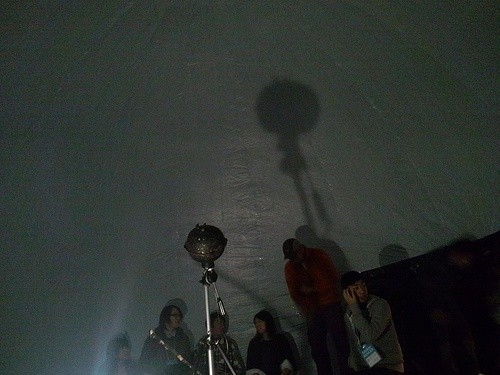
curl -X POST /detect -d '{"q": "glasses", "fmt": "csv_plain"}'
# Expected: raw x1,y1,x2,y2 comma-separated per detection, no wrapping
168,312,183,317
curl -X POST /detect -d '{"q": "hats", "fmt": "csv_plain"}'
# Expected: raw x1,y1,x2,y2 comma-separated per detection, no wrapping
282,238,300,260
339,271,370,289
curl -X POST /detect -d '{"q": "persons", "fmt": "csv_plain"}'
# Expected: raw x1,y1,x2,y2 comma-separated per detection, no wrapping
341,239,500,375
99,335,139,375
246,311,296,375
192,312,243,375
282,238,350,375
139,305,195,375
